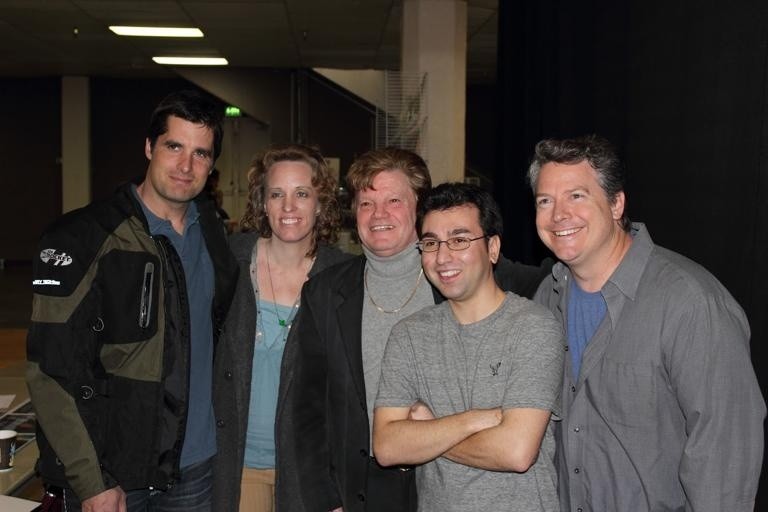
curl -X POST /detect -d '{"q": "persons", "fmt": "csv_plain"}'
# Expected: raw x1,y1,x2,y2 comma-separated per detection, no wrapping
212,139,349,512
274,146,558,512
24,88,242,512
525,134,767,512
371,181,565,511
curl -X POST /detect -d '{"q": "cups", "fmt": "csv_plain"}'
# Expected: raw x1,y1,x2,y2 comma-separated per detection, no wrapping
0,430,15,473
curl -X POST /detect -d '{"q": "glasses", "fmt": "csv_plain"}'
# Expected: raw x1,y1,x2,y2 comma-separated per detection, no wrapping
416,236,484,252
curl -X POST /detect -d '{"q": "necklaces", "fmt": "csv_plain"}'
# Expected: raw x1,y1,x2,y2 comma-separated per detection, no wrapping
263,244,312,327
362,260,423,316
